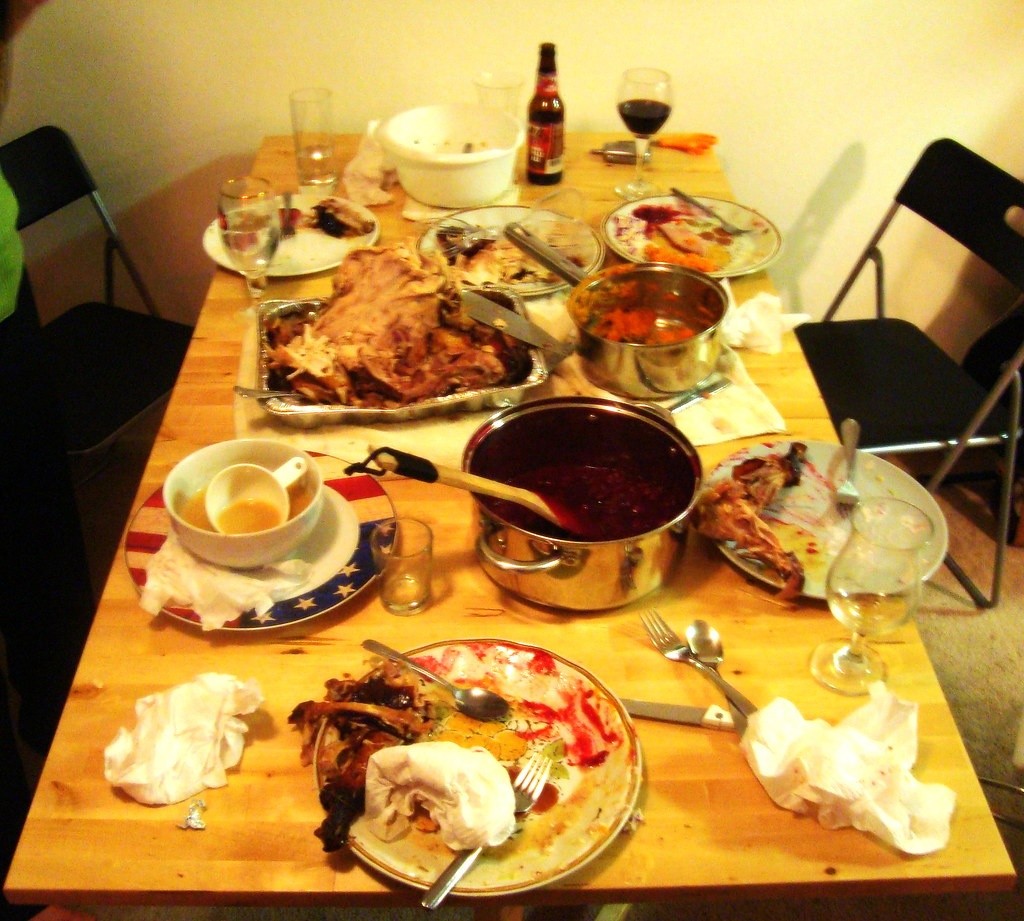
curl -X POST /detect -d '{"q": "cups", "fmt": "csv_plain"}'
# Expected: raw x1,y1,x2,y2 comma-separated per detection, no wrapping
288,87,341,188
370,516,434,617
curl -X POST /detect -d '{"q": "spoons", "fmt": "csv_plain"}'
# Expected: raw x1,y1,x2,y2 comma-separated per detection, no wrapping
360,639,510,722
685,619,749,740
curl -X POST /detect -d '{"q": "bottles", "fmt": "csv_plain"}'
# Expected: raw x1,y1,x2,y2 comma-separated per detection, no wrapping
525,42,567,186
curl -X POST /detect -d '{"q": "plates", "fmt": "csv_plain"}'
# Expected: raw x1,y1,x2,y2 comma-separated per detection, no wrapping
123,449,398,633
201,194,381,277
414,204,605,296
701,440,948,601
597,193,785,278
314,639,644,899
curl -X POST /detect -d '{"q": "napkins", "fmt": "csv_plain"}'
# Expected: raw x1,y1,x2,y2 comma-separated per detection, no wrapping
742,682,958,856
711,276,812,354
364,740,515,852
343,116,398,207
139,533,321,633
100,671,265,806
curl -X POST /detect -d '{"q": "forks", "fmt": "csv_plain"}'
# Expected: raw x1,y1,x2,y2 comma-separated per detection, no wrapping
420,751,553,910
671,187,753,236
833,418,860,521
636,609,758,716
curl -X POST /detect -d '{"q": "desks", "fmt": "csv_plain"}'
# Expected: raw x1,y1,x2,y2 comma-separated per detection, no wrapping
3,132,1017,921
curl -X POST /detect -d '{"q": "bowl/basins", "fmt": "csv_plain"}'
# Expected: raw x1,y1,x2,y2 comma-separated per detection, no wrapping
163,438,325,571
377,102,527,209
205,463,291,533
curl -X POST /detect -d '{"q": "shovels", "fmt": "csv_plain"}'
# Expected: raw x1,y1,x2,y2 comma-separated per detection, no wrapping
367,444,582,533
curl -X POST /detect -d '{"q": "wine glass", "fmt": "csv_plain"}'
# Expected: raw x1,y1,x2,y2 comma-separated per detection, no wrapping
612,66,678,201
810,495,935,696
215,176,282,325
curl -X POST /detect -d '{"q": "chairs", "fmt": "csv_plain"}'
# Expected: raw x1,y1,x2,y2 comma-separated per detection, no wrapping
0,125,193,490
790,140,1024,609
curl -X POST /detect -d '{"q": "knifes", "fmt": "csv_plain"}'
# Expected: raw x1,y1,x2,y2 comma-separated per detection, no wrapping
615,697,740,734
454,282,563,355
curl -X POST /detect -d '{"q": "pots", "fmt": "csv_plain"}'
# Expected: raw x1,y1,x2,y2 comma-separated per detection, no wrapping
460,394,705,614
505,222,729,401
